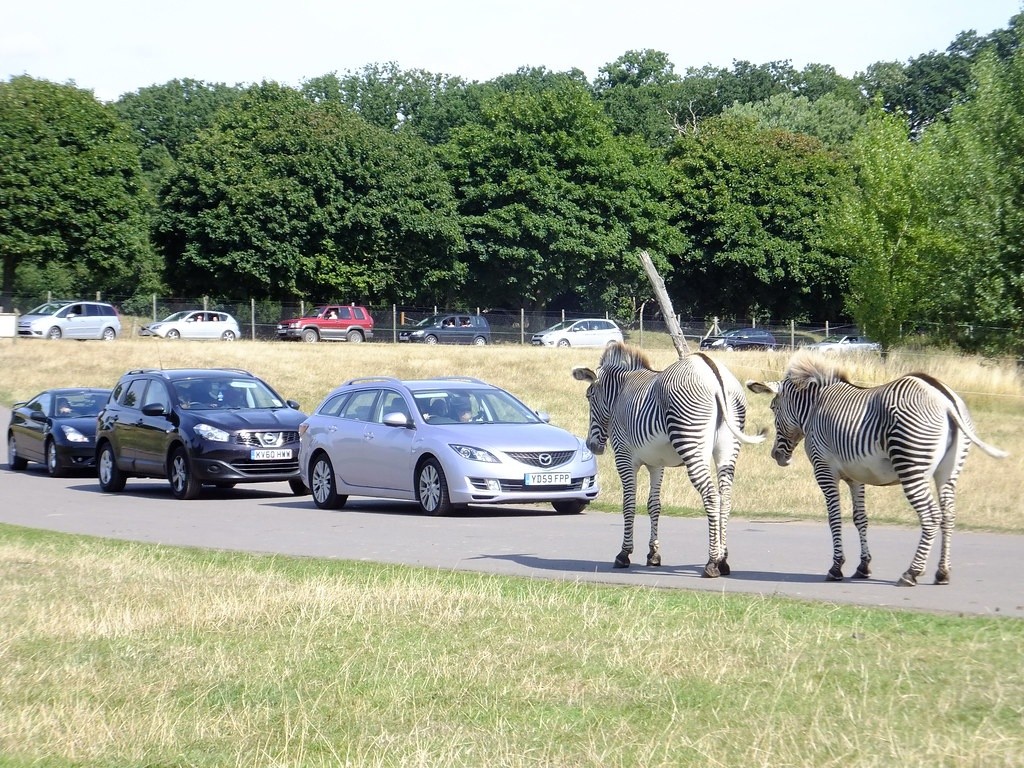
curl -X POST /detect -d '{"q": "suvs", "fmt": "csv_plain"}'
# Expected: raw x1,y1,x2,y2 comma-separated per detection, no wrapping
94,368,312,499
276,304,374,345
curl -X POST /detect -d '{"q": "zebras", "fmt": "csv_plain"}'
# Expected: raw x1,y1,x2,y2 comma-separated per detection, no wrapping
744,341,1009,586
572,342,769,578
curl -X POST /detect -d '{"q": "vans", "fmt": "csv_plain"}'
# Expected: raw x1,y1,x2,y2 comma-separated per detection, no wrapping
397,313,491,346
700,327,775,352
804,335,882,359
19,299,121,342
530,318,625,349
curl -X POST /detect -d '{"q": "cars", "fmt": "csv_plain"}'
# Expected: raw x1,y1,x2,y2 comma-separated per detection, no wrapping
138,311,241,343
297,378,599,517
7,388,114,477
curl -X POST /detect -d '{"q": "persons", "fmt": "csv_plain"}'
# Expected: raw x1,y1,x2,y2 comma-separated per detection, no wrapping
454,397,474,422
178,388,217,408
197,315,202,321
579,323,599,330
852,338,857,342
57,398,71,415
329,310,338,319
213,316,219,321
446,319,471,327
